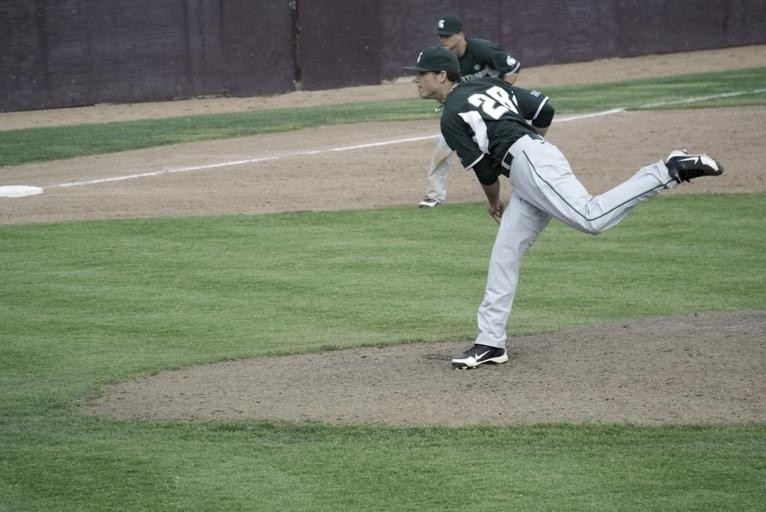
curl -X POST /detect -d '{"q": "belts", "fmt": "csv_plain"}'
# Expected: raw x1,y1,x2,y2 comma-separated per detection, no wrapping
500,132,545,179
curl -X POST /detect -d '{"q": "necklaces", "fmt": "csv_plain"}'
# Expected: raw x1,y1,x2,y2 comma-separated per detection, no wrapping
433,82,459,112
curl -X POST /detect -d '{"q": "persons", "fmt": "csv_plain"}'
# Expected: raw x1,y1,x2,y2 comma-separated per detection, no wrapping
403,43,725,369
418,13,522,210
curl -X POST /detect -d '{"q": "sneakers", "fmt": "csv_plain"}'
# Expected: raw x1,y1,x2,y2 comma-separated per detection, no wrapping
664,149,724,185
451,342,509,370
418,196,439,209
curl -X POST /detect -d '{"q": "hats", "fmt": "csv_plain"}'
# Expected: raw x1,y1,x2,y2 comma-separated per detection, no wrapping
435,15,464,36
402,46,461,74
